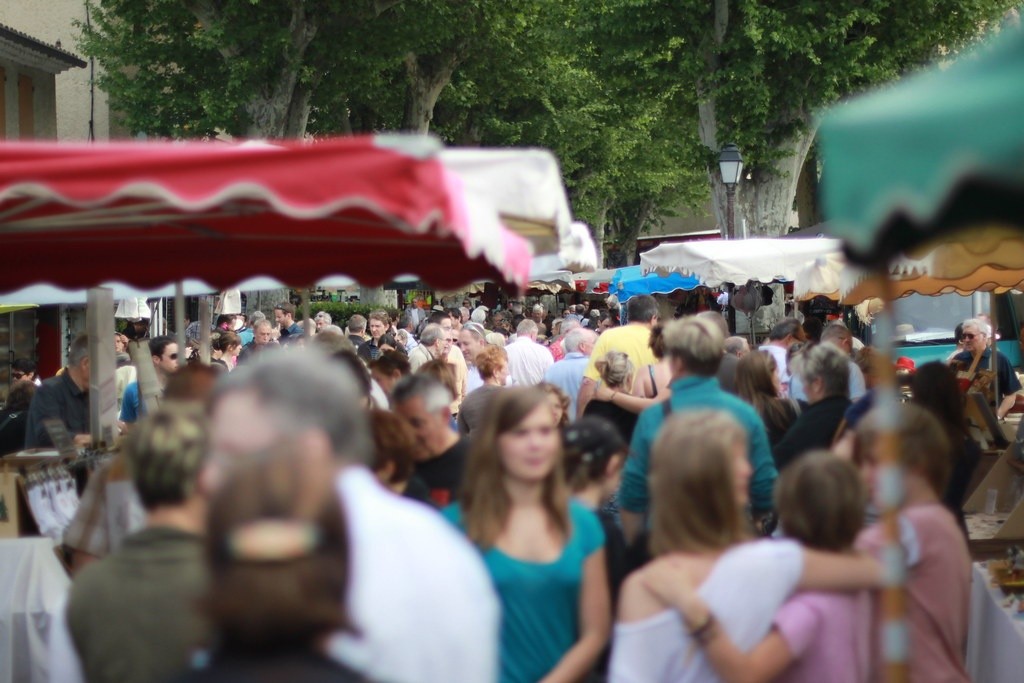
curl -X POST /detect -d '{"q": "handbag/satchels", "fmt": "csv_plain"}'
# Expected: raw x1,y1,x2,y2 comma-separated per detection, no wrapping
964,560,1024,683
955,350,983,394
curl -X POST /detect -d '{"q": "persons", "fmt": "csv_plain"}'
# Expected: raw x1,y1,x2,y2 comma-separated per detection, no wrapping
0,286,1024,683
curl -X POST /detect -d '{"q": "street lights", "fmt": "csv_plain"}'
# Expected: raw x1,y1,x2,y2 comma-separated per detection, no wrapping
718,142,744,238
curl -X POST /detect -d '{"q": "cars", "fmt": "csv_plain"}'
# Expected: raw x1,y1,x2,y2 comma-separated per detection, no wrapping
842,287,1024,370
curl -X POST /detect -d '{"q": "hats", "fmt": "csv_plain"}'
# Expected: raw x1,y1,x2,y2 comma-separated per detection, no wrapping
471,308,485,324
590,309,600,317
203,463,351,626
431,304,443,312
895,357,917,371
464,322,486,337
477,306,490,312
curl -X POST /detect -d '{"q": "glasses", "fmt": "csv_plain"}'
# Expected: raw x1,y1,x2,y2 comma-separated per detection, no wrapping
314,314,323,317
162,353,178,359
273,304,284,309
12,373,26,379
961,333,976,339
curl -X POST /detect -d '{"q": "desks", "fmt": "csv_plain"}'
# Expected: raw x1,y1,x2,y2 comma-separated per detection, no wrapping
964,512,1024,683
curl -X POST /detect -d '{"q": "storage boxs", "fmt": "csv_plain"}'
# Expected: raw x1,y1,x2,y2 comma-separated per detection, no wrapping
962,449,1015,514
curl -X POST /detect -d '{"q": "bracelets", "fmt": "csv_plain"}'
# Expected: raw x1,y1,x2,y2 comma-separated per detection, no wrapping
691,614,713,636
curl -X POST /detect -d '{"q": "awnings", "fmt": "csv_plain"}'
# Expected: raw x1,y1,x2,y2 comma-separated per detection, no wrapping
611,24,1023,332
1,134,594,303
